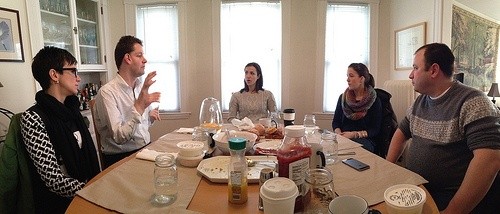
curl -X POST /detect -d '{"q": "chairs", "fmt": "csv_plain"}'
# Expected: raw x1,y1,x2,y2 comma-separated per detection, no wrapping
383,78,417,168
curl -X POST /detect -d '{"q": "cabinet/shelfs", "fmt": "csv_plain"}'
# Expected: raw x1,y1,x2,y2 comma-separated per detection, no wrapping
26,0,108,172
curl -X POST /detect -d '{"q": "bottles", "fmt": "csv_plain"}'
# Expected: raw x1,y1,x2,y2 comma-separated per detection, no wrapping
76,80,104,112
303,114,316,126
321,133,338,167
199,97,223,133
305,125,323,144
228,138,249,207
258,168,274,211
283,108,296,126
276,125,312,213
177,141,204,156
153,154,178,204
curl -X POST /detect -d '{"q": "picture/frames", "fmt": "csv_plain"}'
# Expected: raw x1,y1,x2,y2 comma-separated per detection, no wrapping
0,6,26,62
441,0,500,94
394,21,428,71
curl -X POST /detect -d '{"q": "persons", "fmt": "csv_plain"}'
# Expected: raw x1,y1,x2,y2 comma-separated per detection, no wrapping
332,63,382,154
20,45,101,214
386,43,500,214
228,63,279,125
93,36,160,171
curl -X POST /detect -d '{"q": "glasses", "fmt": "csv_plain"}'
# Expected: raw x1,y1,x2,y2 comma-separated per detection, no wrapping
55,67,78,78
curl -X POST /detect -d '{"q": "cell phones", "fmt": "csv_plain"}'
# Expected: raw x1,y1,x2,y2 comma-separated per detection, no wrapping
341,158,370,172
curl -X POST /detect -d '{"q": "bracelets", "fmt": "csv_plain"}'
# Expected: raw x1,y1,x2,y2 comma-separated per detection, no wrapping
353,131,367,138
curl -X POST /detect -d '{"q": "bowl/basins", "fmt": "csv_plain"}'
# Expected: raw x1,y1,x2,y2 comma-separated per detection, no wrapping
212,130,257,155
178,151,205,167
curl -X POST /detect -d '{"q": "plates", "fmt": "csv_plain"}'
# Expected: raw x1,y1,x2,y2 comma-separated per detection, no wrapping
197,156,279,183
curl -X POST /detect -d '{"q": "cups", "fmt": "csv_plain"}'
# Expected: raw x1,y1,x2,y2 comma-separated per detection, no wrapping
259,117,277,128
192,127,216,159
303,166,335,214
328,194,383,214
260,176,300,214
306,142,326,168
384,184,426,214
40,0,101,66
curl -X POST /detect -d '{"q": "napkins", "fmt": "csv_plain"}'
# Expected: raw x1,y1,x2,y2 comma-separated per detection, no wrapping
136,149,179,162
177,128,194,134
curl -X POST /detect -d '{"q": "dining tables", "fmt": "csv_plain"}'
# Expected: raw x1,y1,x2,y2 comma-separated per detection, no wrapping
65,125,440,214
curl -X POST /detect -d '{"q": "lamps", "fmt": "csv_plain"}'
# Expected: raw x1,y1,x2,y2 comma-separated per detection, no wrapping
486,83,500,105
